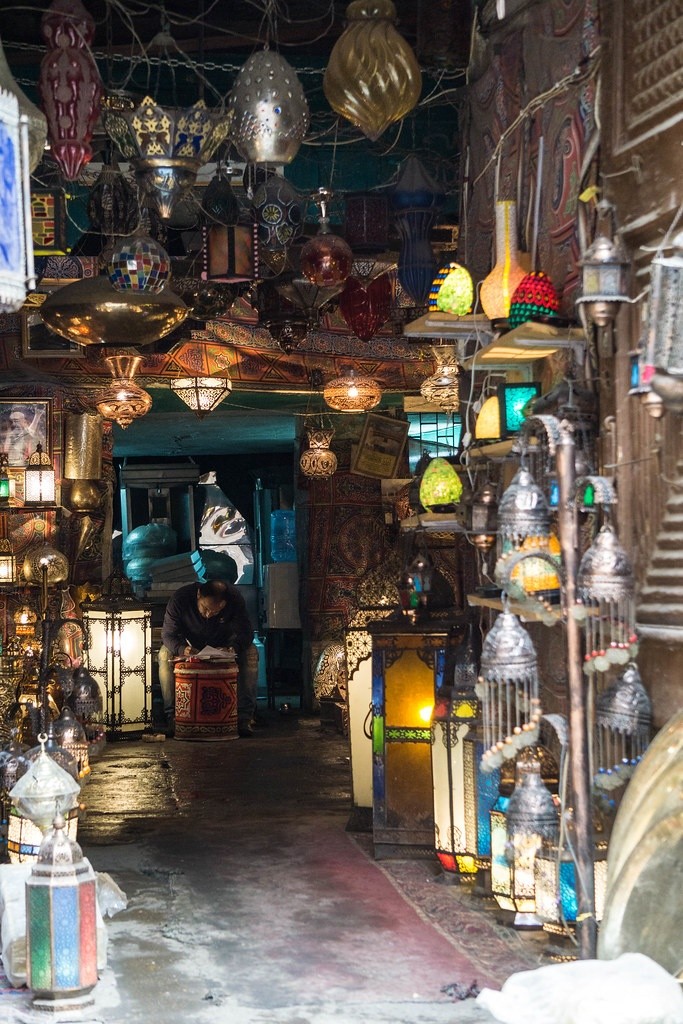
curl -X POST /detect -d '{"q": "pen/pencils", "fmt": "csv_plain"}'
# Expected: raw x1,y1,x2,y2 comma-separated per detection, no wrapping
185,638,192,647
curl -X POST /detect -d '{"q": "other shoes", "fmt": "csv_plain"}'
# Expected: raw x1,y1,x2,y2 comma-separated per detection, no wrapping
237,718,256,738
164,722,175,738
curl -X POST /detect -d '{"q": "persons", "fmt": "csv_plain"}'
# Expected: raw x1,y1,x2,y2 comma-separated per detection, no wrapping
158,576,273,736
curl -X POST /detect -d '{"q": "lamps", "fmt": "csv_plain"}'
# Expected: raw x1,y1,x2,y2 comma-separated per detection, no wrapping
0,0,424,288
299,426,338,480
94,355,153,430
575,198,632,326
23,441,57,507
0,539,70,586
7,602,155,1011
478,200,528,319
419,458,463,512
0,452,11,507
476,396,500,439
312,549,608,956
427,262,475,317
323,369,383,412
171,376,233,420
420,344,459,415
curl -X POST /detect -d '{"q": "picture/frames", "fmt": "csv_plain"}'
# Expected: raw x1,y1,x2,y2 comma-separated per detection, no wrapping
0,397,55,472
